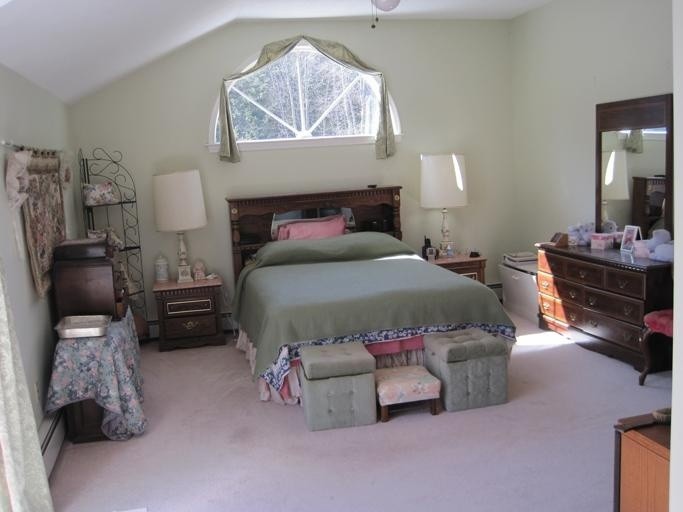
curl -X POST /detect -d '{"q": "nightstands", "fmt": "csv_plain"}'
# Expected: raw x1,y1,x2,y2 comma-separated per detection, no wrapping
151,278,225,352
429,252,487,286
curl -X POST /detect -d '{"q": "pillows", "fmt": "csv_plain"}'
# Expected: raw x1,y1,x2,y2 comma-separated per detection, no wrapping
276,215,346,242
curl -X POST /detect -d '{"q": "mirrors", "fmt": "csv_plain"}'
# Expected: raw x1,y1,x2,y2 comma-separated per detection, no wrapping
594,94,673,250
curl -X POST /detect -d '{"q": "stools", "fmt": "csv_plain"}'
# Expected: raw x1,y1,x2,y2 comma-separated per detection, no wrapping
297,326,508,431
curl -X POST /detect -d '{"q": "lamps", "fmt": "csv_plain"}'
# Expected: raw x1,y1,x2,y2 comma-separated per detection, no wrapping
151,168,206,284
418,152,467,258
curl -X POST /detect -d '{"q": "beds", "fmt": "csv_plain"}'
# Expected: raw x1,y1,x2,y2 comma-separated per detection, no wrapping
224,186,514,401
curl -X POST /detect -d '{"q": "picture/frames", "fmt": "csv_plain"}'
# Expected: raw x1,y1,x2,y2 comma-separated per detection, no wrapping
619,224,638,254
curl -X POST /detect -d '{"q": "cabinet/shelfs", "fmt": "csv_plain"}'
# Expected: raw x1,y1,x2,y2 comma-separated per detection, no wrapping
533,242,673,374
51,258,129,322
77,146,150,346
497,261,538,324
